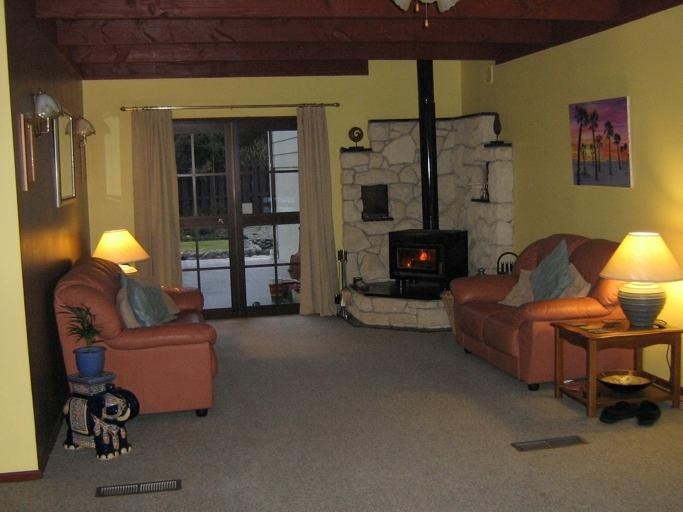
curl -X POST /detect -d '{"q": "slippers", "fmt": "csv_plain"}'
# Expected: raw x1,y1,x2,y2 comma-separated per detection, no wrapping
637,400,661,426
600,400,638,423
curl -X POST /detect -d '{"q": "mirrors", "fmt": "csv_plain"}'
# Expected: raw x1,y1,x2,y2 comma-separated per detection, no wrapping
52,105,78,209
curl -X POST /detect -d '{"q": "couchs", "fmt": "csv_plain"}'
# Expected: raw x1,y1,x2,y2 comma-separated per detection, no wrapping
51,254,220,418
449,233,635,391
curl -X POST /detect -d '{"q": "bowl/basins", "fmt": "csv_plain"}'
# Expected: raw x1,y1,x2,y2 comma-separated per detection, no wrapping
597,371,656,392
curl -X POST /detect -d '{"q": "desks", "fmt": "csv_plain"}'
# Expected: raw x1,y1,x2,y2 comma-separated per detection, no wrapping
549,318,683,417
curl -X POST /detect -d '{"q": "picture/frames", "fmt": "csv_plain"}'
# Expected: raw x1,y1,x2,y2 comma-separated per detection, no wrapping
20,112,37,191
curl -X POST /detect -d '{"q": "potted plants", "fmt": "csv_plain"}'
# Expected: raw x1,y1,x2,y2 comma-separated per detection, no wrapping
53,302,107,377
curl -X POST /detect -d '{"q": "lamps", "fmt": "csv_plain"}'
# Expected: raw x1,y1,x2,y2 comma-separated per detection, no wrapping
598,231,683,328
29,86,96,148
92,228,152,276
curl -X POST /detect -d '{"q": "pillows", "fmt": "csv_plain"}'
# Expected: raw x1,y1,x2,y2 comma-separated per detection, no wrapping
115,272,180,329
498,238,592,307
